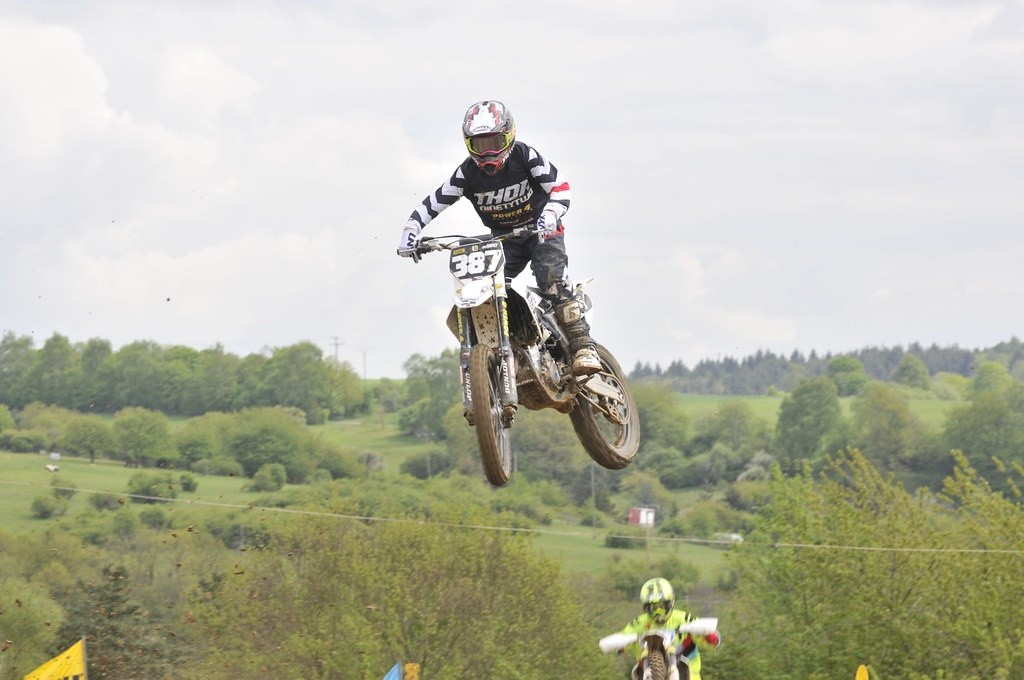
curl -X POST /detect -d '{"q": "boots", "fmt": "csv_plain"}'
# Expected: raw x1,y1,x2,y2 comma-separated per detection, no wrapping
553,278,604,376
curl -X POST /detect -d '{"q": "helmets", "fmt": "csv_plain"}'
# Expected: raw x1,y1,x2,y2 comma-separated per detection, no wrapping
640,577,675,623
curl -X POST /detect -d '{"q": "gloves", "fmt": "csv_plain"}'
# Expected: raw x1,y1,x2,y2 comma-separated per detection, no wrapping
398,229,422,263
537,211,557,243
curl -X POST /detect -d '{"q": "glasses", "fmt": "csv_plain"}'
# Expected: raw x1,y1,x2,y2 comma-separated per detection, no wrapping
466,133,508,156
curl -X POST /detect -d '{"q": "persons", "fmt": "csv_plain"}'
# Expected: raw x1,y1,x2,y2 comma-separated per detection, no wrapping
613,577,721,680
398,100,602,375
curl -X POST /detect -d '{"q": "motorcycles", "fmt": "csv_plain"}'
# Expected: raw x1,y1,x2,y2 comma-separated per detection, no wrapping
396,224,642,486
598,618,719,680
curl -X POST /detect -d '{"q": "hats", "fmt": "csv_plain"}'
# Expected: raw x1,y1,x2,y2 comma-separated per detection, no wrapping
462,100,516,177
